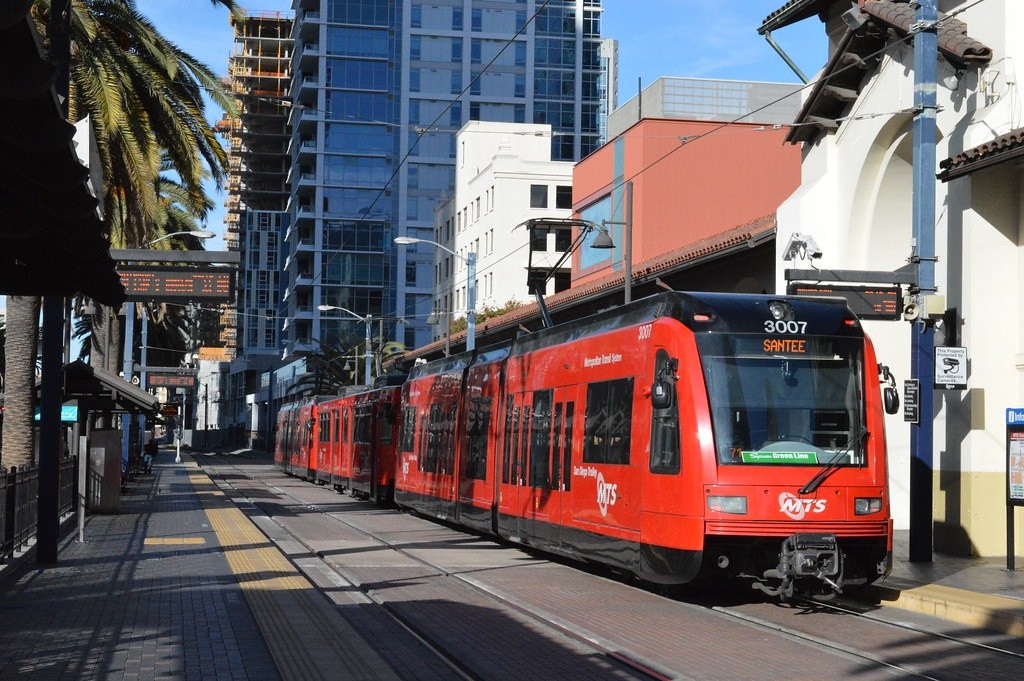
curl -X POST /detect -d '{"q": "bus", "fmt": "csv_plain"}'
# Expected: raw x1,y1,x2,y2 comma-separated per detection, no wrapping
273,291,900,603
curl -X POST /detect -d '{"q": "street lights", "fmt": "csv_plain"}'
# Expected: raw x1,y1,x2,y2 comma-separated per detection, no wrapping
119,230,216,475
316,305,372,386
394,237,477,352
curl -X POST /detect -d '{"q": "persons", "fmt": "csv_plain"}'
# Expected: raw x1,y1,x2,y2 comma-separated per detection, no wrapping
141,449,156,476
144,425,154,453
